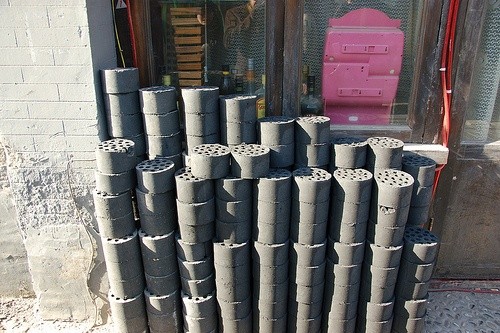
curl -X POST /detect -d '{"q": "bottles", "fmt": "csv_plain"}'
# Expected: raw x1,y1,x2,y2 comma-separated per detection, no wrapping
301,75,323,118
254,75,266,121
245,58,256,96
161,72,172,87
301,65,309,96
219,64,233,94
231,68,243,94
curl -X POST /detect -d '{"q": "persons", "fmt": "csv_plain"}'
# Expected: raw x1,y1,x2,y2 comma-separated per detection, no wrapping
223,1,264,80
